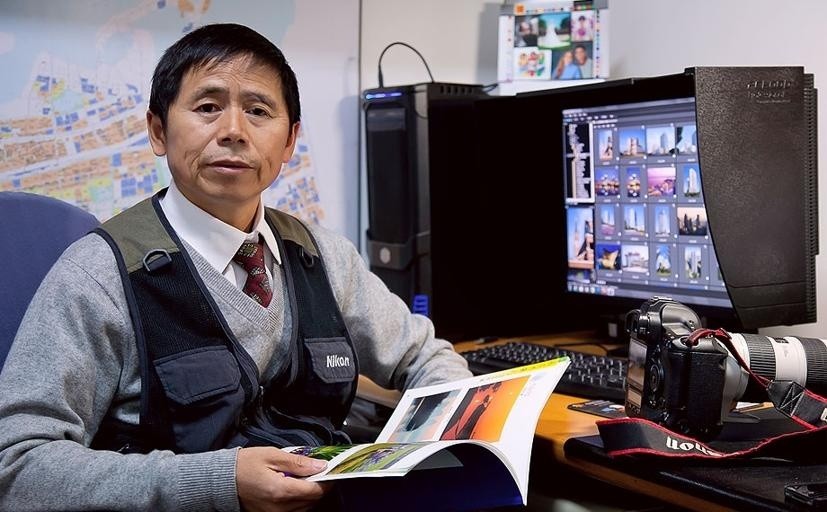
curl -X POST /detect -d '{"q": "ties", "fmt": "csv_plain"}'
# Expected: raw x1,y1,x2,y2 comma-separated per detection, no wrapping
232,242,273,307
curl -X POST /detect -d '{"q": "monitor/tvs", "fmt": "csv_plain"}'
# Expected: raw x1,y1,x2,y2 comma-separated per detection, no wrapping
561,98,728,329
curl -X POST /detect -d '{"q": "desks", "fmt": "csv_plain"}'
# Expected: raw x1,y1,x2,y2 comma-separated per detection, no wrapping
349,307,827,512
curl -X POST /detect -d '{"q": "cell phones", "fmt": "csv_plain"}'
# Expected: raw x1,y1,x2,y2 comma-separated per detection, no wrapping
784,481,827,506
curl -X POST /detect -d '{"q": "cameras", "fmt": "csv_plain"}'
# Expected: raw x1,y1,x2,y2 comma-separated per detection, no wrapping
624,299,827,443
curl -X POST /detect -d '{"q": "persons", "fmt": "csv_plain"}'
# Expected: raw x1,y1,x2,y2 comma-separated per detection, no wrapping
534,53,545,79
571,16,594,44
516,50,527,78
0,21,477,509
526,52,536,78
571,46,591,78
517,22,537,46
551,49,581,79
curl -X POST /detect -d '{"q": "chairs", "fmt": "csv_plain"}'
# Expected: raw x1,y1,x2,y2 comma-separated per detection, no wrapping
0,188,104,385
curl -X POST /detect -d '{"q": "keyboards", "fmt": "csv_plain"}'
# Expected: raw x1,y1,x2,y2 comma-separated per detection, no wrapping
459,336,628,405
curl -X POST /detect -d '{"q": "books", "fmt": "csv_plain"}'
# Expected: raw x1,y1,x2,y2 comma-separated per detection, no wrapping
267,351,571,512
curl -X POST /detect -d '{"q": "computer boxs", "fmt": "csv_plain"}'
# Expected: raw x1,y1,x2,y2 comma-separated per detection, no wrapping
360,83,491,343
